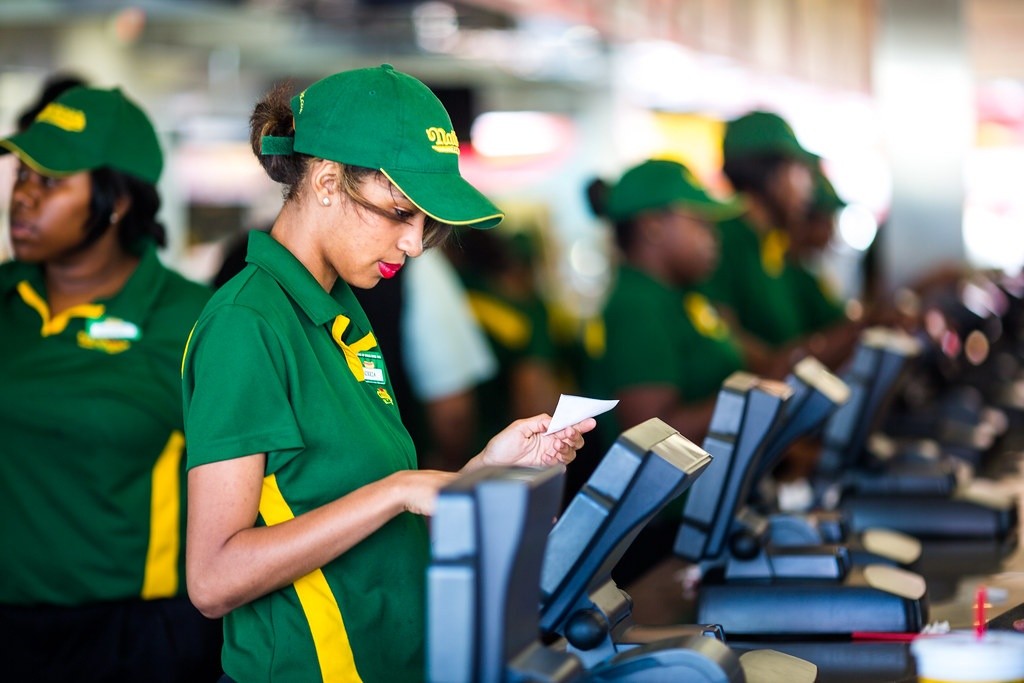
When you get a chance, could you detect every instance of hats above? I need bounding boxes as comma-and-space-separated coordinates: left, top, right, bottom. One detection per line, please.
723, 114, 819, 178
812, 177, 846, 211
603, 159, 748, 226
0, 88, 164, 183
260, 64, 504, 230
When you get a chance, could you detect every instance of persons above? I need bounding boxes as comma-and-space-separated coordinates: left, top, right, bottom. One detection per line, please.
0, 72, 212, 683
177, 64, 597, 683
585, 110, 914, 441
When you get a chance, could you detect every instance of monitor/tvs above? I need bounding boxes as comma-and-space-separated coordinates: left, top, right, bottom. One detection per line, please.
425, 282, 1008, 683
